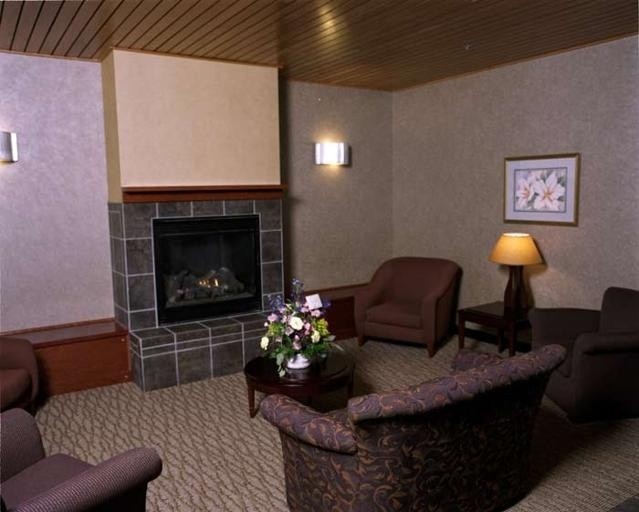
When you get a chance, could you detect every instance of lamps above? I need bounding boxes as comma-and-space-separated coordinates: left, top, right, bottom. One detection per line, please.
315, 142, 349, 165
489, 232, 545, 313
0, 131, 18, 162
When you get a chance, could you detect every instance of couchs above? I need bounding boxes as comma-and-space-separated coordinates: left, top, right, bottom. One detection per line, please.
259, 343, 568, 512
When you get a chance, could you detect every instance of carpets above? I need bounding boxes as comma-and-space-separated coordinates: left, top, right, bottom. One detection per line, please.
26, 334, 639, 512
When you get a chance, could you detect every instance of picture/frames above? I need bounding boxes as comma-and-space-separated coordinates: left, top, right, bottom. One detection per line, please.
503, 152, 580, 226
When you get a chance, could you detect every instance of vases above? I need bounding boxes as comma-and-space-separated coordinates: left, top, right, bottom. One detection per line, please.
284, 352, 312, 370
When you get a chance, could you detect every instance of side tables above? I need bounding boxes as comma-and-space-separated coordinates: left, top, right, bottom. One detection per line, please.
457, 300, 533, 357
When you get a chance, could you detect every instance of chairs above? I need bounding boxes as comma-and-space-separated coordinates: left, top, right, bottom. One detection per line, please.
0, 334, 39, 414
527, 286, 639, 423
0, 408, 162, 512
354, 256, 461, 357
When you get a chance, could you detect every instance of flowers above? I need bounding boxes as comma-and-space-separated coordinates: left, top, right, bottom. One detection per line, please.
260, 277, 336, 379
